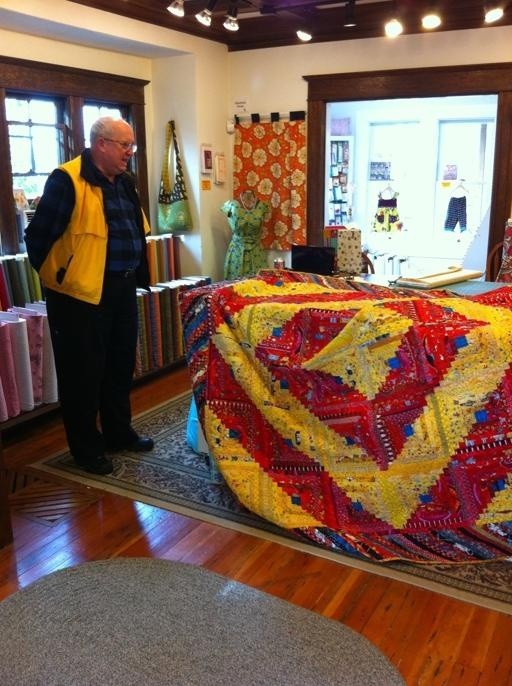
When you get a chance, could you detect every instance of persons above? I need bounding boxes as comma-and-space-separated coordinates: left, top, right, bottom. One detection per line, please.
23, 115, 154, 476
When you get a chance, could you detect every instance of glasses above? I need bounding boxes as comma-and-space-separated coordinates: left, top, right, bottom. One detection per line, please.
105, 138, 138, 152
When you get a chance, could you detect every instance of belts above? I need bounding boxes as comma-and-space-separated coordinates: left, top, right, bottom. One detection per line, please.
105, 268, 132, 280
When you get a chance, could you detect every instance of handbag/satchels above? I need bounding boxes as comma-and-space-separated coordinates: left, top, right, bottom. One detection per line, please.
157, 191, 193, 233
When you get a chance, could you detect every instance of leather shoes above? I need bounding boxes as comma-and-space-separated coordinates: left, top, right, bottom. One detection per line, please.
70, 436, 154, 474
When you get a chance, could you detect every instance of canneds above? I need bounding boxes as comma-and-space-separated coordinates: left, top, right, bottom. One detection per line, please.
273, 259, 285, 269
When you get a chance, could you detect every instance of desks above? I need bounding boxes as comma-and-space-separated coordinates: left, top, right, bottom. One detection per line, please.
178, 266, 512, 499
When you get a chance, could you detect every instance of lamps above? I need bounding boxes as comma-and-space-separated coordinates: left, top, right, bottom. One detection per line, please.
291, 1, 506, 43
165, 0, 244, 32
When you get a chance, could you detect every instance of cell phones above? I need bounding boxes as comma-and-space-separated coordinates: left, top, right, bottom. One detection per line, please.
56, 268, 66, 284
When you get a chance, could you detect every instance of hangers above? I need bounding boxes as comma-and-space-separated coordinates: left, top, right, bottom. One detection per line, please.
378, 180, 400, 199
449, 179, 469, 197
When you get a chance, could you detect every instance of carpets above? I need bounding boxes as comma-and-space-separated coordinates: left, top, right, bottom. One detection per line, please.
1, 556, 410, 686
27, 387, 511, 620
